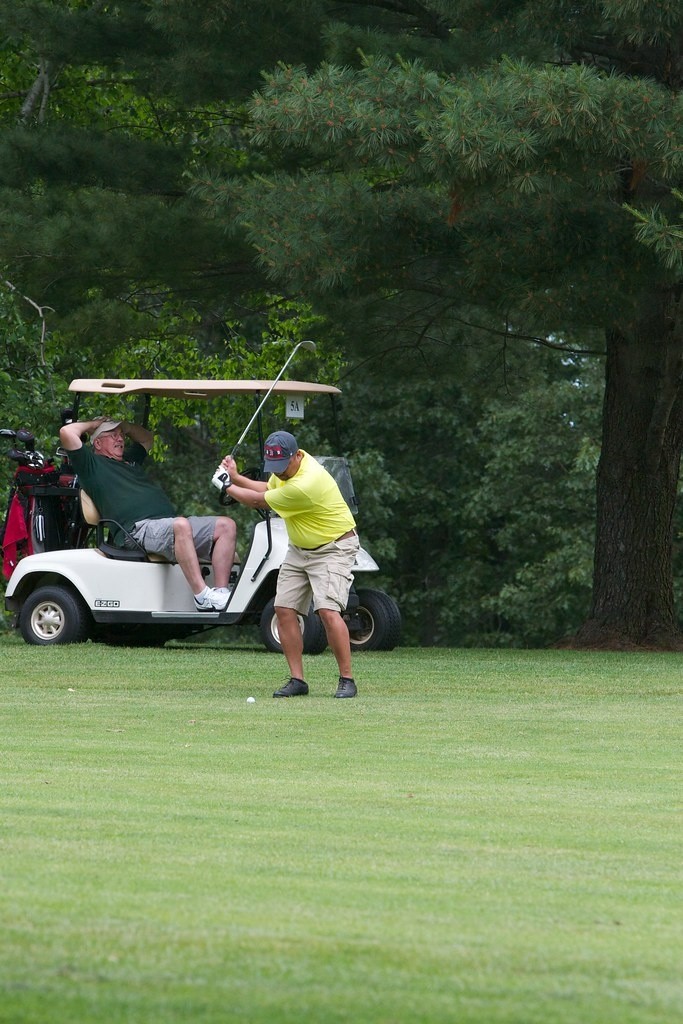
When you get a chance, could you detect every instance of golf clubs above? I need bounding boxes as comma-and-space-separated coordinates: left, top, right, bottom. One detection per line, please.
217, 340, 315, 486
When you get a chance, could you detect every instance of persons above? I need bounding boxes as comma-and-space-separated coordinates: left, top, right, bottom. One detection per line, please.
60, 416, 237, 612
211, 431, 361, 698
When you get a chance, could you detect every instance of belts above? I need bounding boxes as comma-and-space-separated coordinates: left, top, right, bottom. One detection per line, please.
335, 530, 356, 542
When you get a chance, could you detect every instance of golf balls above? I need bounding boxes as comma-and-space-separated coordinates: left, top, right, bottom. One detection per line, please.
247, 697, 256, 703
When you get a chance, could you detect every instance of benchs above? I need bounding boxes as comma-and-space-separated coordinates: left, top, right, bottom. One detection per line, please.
79, 488, 242, 566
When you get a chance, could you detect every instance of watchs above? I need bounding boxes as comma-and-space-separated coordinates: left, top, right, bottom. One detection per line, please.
222, 481, 232, 493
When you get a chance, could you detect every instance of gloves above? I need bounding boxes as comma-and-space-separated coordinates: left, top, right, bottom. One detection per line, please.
211, 465, 230, 491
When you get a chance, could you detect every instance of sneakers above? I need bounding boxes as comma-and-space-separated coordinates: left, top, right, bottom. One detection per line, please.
193, 586, 231, 612
272, 677, 309, 698
333, 674, 357, 698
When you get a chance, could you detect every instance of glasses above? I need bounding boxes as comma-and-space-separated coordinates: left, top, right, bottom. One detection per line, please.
97, 432, 125, 441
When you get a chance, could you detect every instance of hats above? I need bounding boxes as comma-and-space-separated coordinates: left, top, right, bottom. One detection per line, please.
89, 416, 122, 445
263, 430, 299, 474
